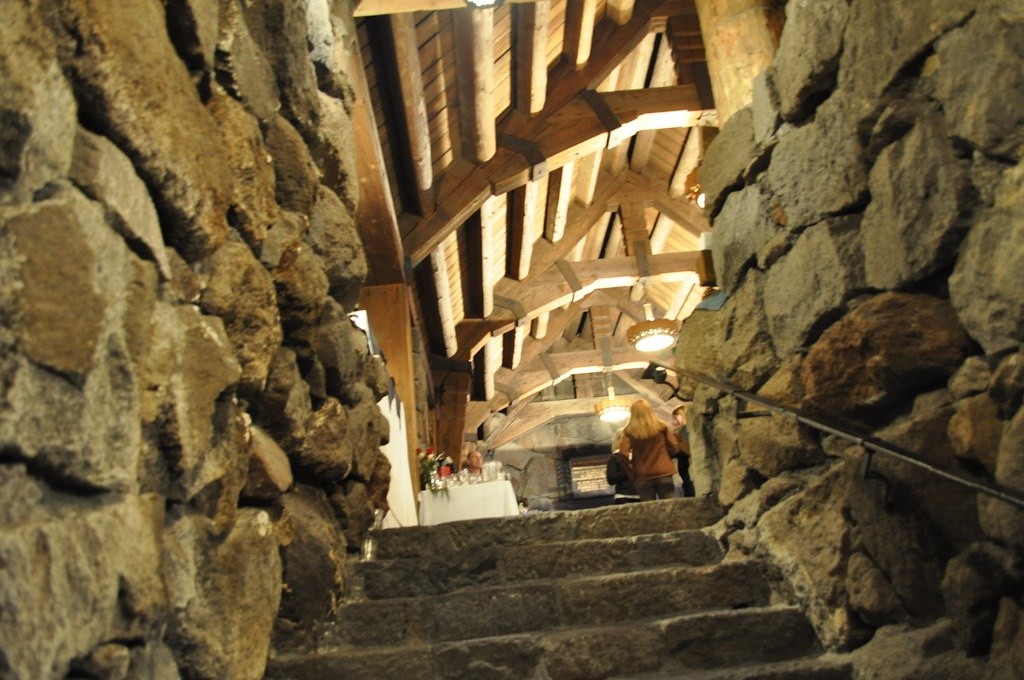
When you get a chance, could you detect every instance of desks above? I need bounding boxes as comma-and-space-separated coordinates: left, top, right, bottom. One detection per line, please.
419, 480, 519, 526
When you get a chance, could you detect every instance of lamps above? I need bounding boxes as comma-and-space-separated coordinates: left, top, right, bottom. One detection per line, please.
594, 371, 633, 422
627, 282, 679, 353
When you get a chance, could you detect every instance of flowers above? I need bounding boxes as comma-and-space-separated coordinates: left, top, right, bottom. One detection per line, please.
416, 448, 454, 476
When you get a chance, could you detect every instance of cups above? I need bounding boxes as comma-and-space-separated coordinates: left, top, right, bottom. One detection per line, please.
374, 509, 383, 528
360, 539, 377, 561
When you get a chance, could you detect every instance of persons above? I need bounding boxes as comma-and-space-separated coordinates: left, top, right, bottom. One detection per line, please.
467, 451, 483, 474
671, 406, 696, 497
606, 427, 641, 504
621, 400, 679, 503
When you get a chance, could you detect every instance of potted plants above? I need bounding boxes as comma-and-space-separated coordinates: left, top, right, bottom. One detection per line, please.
423, 471, 442, 490
